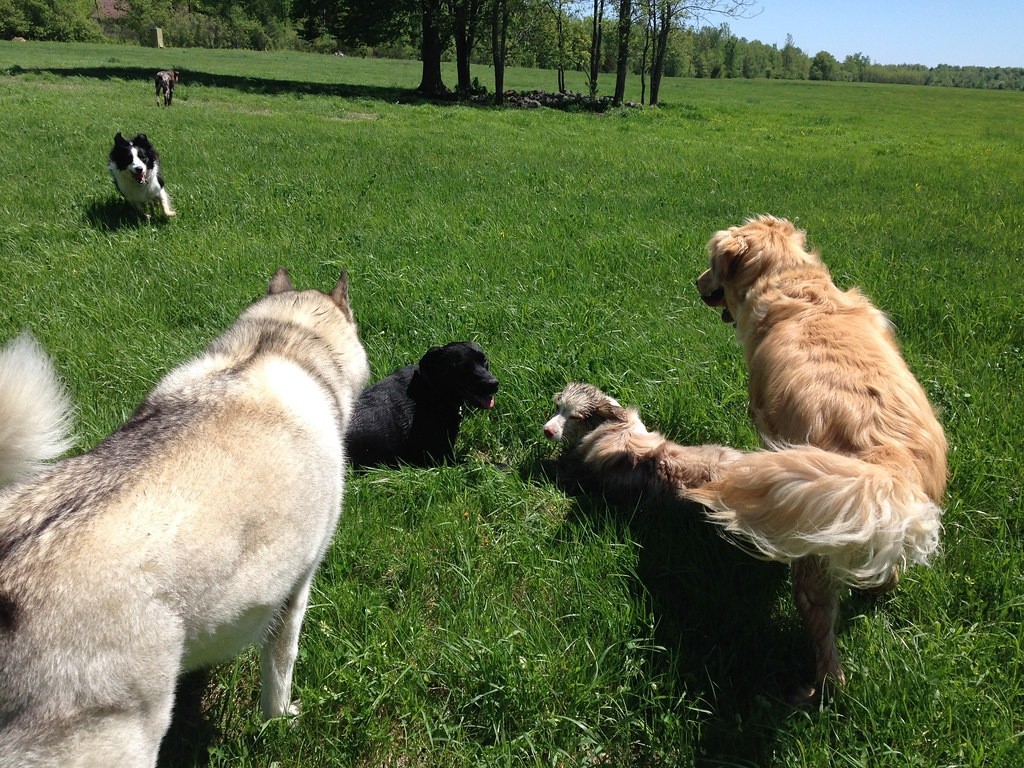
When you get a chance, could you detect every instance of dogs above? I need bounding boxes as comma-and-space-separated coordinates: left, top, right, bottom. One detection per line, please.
542, 212, 953, 707
0, 267, 373, 768
152, 65, 181, 107
107, 131, 177, 227
342, 340, 500, 476
